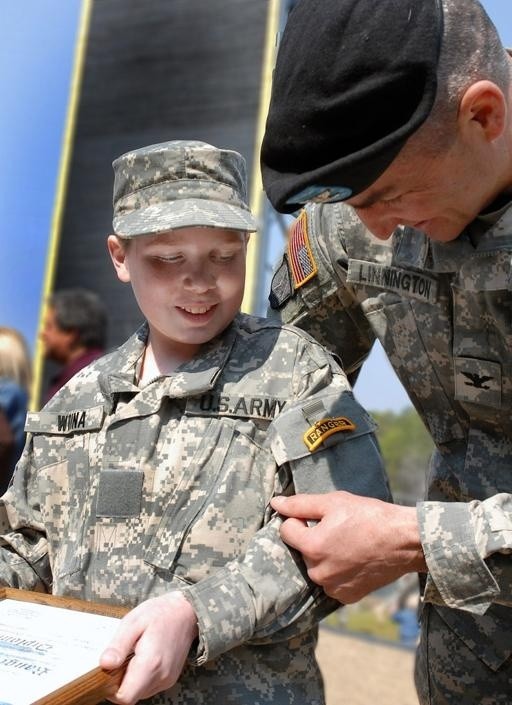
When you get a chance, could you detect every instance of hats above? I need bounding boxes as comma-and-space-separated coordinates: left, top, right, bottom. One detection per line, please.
259, 0, 445, 214
111, 139, 258, 242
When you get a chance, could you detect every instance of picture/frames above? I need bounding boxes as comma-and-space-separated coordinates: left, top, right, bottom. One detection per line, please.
0, 586, 135, 705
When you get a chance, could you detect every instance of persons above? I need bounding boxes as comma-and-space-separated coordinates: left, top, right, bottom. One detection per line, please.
0, 140, 392, 705
0, 288, 111, 489
261, 0, 512, 705
